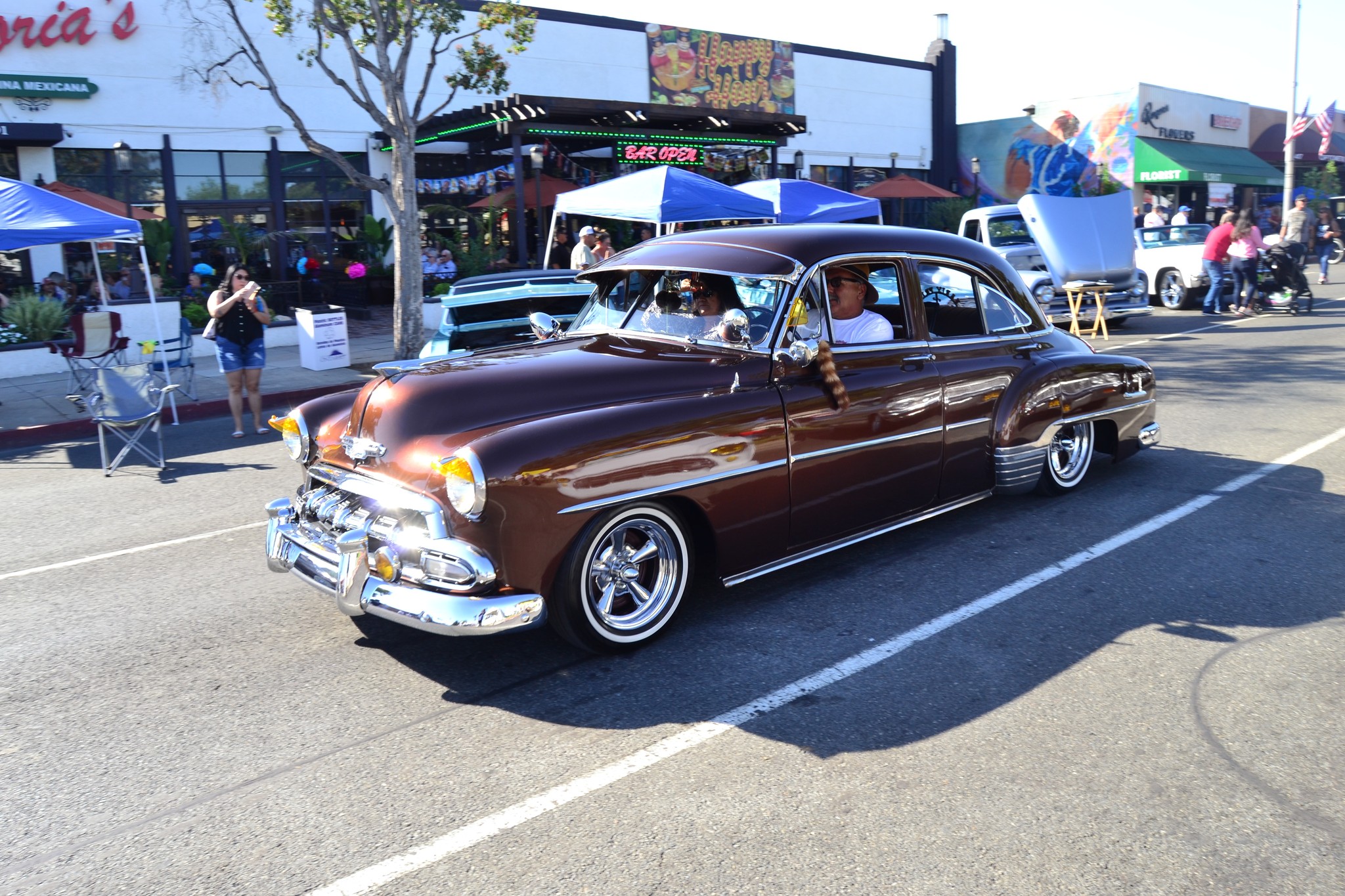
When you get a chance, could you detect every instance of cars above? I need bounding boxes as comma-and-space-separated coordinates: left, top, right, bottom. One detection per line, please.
932, 190, 1154, 327
268, 222, 1159, 661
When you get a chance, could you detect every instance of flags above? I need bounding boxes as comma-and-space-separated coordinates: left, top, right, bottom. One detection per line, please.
416, 138, 608, 194
1315, 100, 1335, 156
1283, 97, 1310, 144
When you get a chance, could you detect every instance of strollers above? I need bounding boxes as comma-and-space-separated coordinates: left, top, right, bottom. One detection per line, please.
1254, 239, 1312, 316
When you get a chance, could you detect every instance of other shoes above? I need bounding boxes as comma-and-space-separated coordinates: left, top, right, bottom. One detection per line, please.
1247, 308, 1261, 313
1317, 278, 1329, 284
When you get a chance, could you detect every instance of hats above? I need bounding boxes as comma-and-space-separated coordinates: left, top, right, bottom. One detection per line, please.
1178, 206, 1192, 211
834, 263, 879, 305
39, 277, 56, 287
1295, 194, 1308, 201
578, 226, 601, 237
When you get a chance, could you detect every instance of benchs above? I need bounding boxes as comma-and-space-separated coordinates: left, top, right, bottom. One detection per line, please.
871, 301, 1009, 339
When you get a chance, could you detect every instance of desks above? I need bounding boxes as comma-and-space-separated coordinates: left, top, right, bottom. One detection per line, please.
1062, 284, 1116, 341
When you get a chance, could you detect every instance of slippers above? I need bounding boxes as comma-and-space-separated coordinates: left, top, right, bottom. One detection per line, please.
232, 431, 245, 438
256, 428, 270, 434
1237, 310, 1254, 317
1228, 305, 1238, 312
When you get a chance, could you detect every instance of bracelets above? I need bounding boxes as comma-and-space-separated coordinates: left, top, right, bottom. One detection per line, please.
247, 305, 258, 313
1280, 237, 1284, 239
1309, 237, 1316, 239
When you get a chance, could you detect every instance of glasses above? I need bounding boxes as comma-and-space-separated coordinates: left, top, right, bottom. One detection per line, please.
825, 277, 862, 288
1318, 211, 1327, 214
120, 273, 131, 277
441, 255, 449, 257
1298, 200, 1309, 202
693, 287, 719, 299
588, 233, 597, 238
232, 273, 250, 281
427, 256, 432, 258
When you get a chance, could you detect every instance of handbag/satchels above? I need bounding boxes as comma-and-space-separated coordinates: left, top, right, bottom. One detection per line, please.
202, 317, 221, 341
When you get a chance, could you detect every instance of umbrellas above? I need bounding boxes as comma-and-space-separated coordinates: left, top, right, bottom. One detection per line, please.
469, 172, 583, 247
37, 181, 167, 305
852, 173, 962, 226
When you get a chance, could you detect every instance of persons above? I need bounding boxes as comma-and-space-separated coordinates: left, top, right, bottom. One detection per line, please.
41, 259, 211, 337
206, 262, 270, 438
423, 194, 1345, 349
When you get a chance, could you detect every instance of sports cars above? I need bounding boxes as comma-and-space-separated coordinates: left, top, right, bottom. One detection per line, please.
1133, 224, 1274, 310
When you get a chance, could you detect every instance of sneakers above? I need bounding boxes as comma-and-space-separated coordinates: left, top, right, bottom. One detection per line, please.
1201, 310, 1223, 317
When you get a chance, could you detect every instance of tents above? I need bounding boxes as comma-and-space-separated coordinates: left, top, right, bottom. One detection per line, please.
0, 175, 180, 426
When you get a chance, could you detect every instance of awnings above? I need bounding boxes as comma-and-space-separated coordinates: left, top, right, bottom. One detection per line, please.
1133, 135, 1284, 187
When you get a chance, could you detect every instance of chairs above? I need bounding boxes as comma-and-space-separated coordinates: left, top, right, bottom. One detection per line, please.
43, 311, 132, 395
84, 363, 178, 479
138, 317, 198, 403
